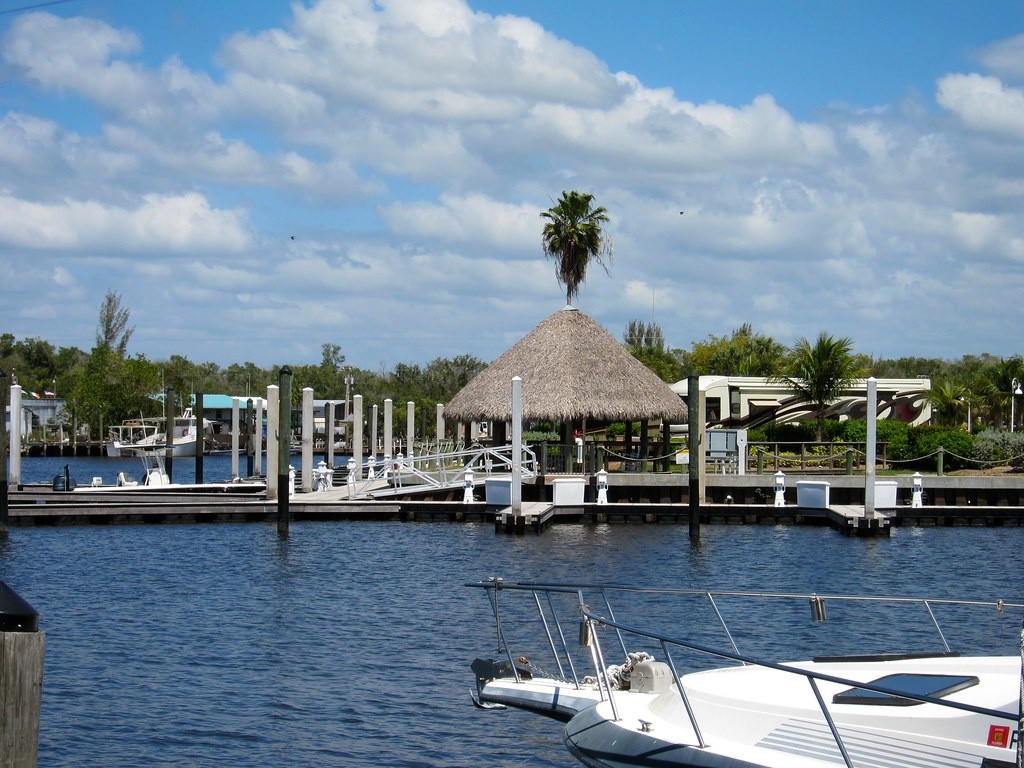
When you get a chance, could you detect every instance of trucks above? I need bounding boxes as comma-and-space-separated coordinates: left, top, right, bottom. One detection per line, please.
659, 375, 931, 438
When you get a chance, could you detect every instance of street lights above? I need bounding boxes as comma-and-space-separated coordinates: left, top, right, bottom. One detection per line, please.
1011, 377, 1023, 433
960, 388, 971, 433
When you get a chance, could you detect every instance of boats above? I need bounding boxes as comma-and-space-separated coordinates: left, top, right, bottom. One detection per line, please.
113, 408, 210, 457
72, 441, 267, 494
465, 576, 1024, 768
